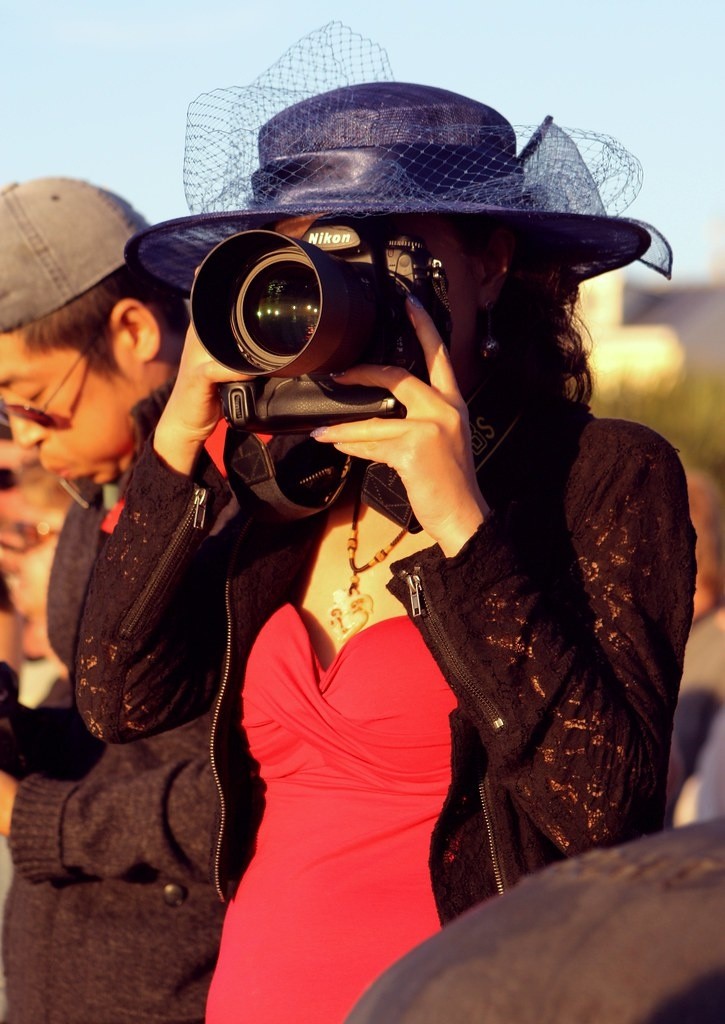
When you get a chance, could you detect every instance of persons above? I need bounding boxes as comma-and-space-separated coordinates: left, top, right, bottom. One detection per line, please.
74, 21, 699, 1023
0, 177, 725, 1024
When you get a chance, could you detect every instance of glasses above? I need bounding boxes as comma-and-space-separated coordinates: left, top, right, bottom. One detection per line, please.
0, 319, 108, 427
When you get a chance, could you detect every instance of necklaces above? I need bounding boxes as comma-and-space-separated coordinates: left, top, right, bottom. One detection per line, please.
323, 492, 407, 642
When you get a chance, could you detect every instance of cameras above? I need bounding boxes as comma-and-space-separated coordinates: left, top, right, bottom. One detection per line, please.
190, 227, 449, 400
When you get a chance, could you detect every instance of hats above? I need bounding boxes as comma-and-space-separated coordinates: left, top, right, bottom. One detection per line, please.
124, 84, 673, 296
0, 178, 151, 331
